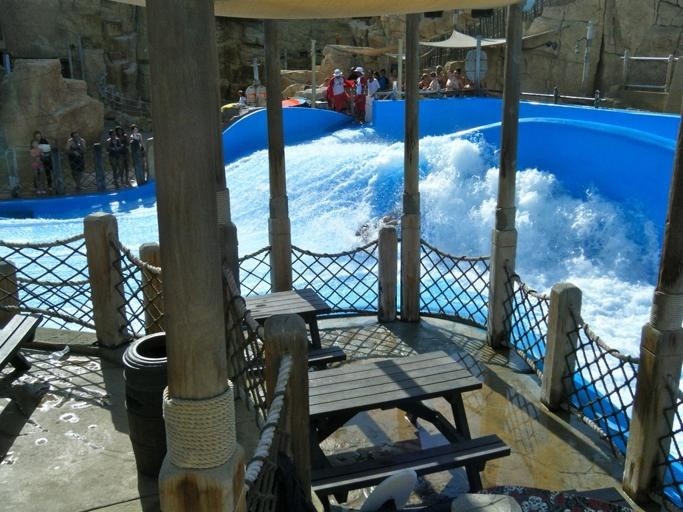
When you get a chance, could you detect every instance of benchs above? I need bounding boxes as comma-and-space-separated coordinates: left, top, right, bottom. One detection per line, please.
247, 349, 344, 380
310, 433, 511, 502
0, 313, 44, 372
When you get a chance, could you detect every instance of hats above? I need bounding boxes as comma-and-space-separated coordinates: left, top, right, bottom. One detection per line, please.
354, 67, 365, 75
333, 69, 342, 76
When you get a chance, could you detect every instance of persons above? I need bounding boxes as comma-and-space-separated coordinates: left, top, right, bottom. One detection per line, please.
128, 123, 147, 186
29, 139, 48, 195
323, 65, 390, 124
105, 128, 125, 188
31, 130, 54, 191
64, 129, 86, 190
418, 64, 474, 98
114, 125, 129, 186
238, 89, 247, 106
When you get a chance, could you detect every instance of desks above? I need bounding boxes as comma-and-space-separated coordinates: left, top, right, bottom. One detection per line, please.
243, 284, 332, 349
300, 351, 482, 455
